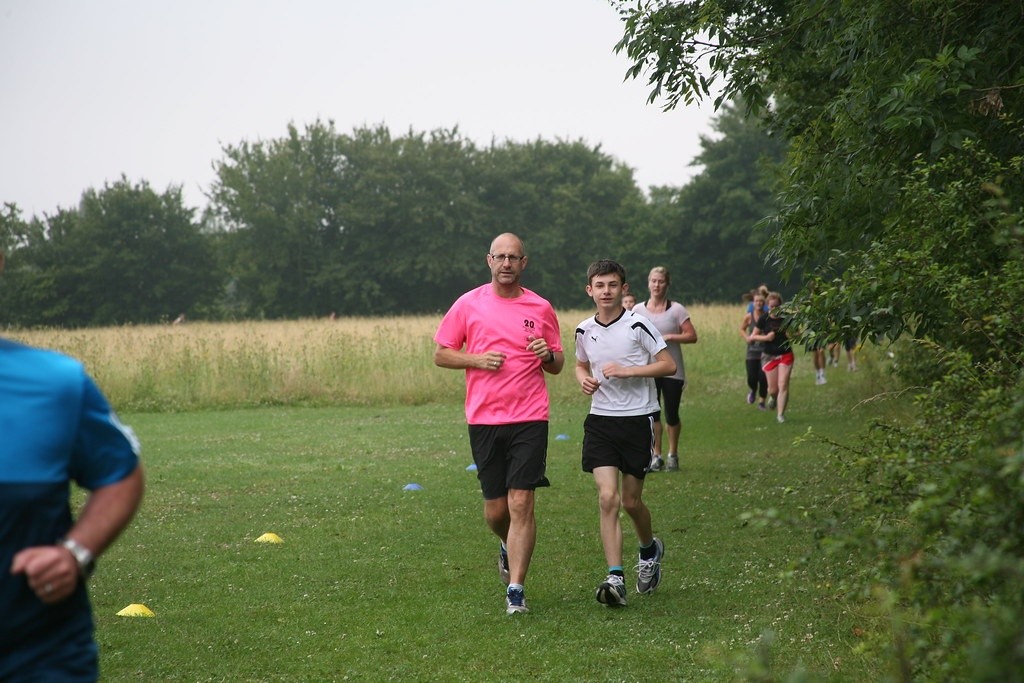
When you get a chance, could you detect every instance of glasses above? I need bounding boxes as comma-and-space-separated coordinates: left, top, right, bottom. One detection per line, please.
490, 254, 525, 263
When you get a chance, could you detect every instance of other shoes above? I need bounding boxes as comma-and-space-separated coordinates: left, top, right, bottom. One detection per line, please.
815, 374, 828, 385
777, 413, 787, 423
846, 362, 859, 373
766, 396, 777, 408
747, 386, 757, 404
827, 357, 838, 367
757, 401, 768, 412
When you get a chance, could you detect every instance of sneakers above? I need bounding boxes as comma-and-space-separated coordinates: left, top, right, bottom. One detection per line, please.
634, 536, 666, 596
503, 583, 530, 616
595, 573, 627, 608
663, 454, 679, 470
497, 541, 512, 587
650, 456, 666, 472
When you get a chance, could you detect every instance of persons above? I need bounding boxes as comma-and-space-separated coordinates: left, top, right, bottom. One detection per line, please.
844, 336, 857, 371
739, 294, 768, 411
632, 267, 697, 472
750, 292, 799, 423
0, 337, 145, 683
621, 293, 635, 311
432, 233, 565, 614
827, 342, 842, 368
813, 347, 828, 386
748, 286, 769, 313
573, 259, 677, 608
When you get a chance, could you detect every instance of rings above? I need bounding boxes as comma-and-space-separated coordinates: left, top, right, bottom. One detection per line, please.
45, 583, 53, 595
494, 362, 496, 365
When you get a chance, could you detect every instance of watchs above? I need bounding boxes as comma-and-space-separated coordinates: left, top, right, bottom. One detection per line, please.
61, 538, 95, 579
542, 350, 555, 363
